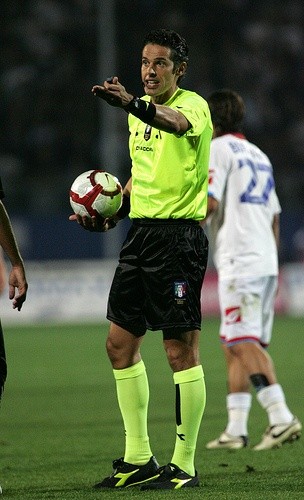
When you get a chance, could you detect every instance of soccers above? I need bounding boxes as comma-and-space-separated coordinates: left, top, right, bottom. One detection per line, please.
70, 169, 123, 222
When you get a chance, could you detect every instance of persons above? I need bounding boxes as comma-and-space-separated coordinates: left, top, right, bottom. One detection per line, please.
0, 179, 28, 495
69, 30, 213, 490
200, 90, 302, 451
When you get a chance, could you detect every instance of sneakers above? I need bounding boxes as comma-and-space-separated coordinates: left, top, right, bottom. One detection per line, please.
92, 456, 163, 489
253, 416, 301, 451
206, 432, 248, 450
140, 463, 200, 489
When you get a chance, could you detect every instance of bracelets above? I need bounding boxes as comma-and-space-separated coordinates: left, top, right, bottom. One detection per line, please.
125, 97, 156, 124
